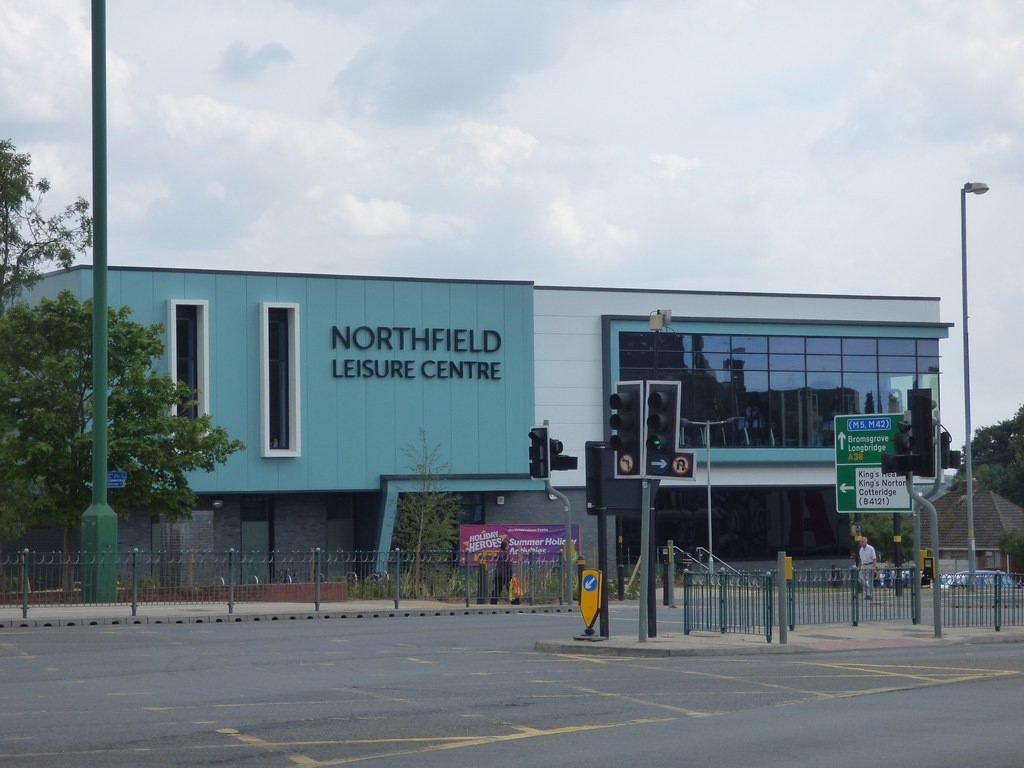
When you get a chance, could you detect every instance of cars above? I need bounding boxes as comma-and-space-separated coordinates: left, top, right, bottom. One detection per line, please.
868, 570, 1021, 588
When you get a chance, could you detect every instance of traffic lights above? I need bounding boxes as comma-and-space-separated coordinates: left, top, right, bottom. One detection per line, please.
607, 380, 642, 478
644, 379, 680, 477
527, 426, 551, 480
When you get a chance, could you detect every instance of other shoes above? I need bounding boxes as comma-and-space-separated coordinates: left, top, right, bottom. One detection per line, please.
865, 596, 872, 600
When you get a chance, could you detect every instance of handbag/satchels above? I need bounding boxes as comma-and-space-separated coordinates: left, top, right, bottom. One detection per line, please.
508, 579, 516, 602
513, 577, 524, 598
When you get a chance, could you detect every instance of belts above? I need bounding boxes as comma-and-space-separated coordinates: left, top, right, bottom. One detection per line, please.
864, 563, 873, 565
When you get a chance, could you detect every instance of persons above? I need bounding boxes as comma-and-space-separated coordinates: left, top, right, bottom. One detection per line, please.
831, 565, 845, 587
873, 565, 892, 589
490, 541, 521, 605
854, 537, 877, 600
